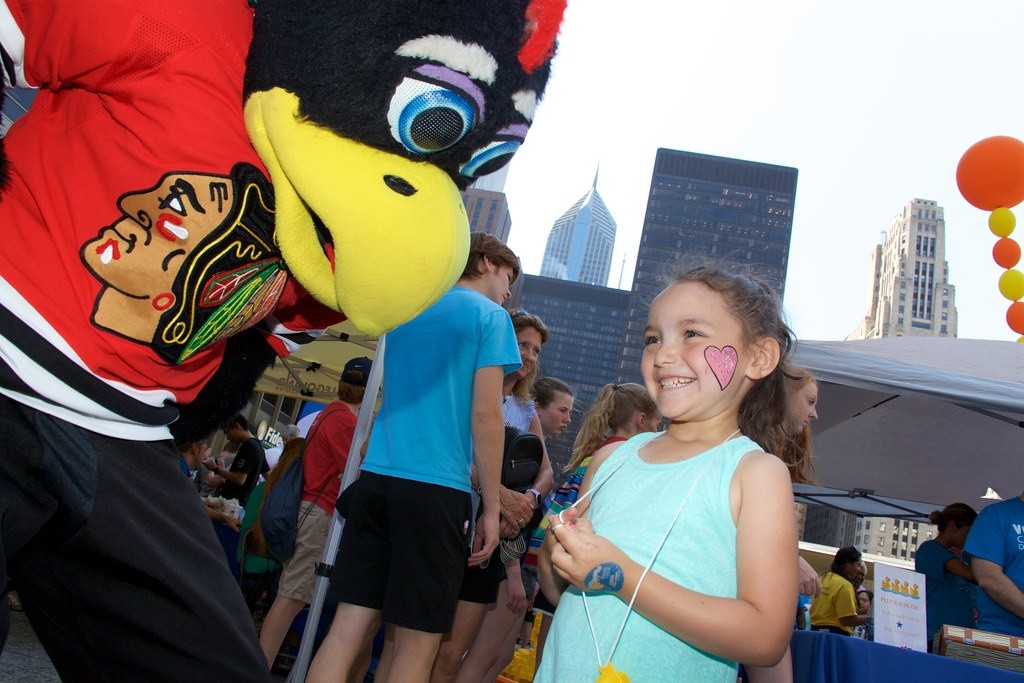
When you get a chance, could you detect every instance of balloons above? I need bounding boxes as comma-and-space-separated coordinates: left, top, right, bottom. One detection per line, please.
956, 135, 1024, 342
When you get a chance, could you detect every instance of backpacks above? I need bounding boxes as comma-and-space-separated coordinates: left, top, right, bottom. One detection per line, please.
480, 425, 544, 569
260, 408, 349, 563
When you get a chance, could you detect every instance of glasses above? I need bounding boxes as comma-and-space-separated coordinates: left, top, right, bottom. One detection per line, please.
511, 308, 534, 319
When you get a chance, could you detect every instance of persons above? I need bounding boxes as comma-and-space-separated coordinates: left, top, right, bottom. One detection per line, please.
809, 494, 1024, 652
184, 231, 822, 683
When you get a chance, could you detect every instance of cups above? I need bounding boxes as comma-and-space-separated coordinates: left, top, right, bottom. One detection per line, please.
228, 504, 244, 520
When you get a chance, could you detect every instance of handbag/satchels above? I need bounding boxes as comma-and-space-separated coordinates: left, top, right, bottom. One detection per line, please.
533, 456, 592, 539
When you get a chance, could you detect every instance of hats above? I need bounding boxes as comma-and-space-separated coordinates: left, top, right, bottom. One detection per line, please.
341, 357, 381, 391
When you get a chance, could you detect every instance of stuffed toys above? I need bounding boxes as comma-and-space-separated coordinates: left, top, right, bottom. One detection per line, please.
0, 0, 562, 683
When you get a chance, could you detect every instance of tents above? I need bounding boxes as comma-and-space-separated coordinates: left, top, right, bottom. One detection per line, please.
253, 320, 382, 683
783, 336, 1024, 524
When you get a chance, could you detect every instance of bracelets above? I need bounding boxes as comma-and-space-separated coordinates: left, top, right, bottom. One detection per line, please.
214, 466, 219, 475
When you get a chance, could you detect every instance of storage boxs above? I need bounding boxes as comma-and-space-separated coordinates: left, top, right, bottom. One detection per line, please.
937, 625, 1024, 674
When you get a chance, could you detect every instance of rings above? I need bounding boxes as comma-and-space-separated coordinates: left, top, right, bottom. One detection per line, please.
512, 528, 517, 534
518, 518, 524, 522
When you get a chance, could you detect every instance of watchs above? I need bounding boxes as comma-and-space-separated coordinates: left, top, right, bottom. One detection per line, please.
526, 488, 542, 509
